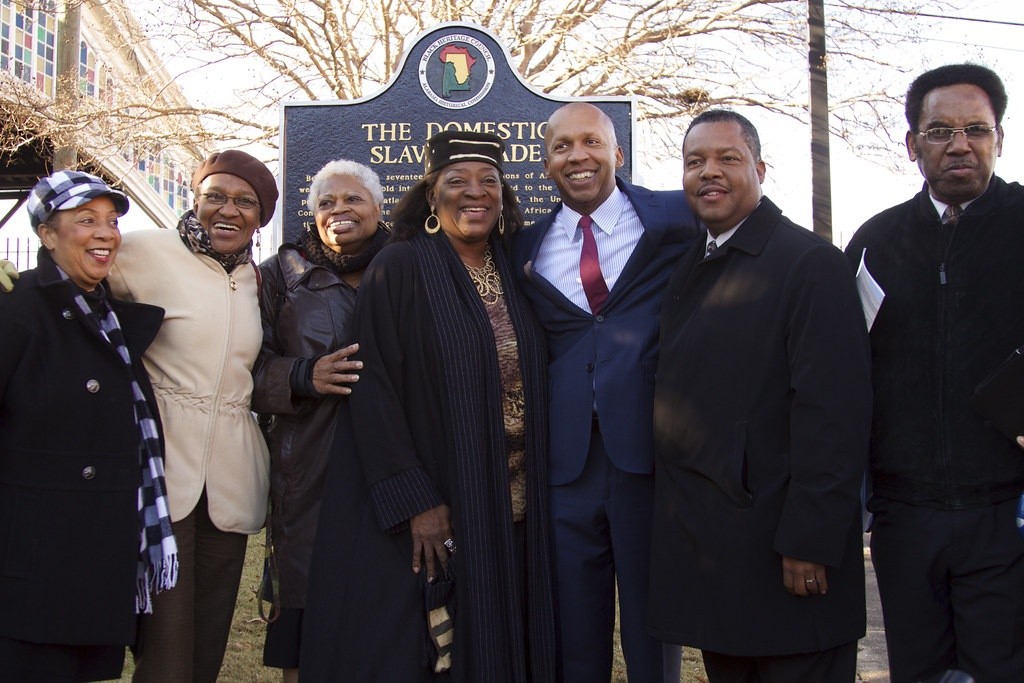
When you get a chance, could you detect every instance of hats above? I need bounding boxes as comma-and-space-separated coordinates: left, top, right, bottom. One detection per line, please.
191, 150, 279, 227
424, 130, 505, 175
26, 170, 129, 240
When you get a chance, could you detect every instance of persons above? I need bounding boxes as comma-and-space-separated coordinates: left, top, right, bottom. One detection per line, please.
503, 103, 701, 682
298, 134, 555, 683
249, 159, 393, 683
647, 109, 875, 683
0, 172, 166, 683
844, 65, 1024, 683
0, 151, 270, 683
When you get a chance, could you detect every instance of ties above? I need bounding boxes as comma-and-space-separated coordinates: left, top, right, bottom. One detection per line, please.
942, 206, 962, 226
704, 241, 715, 258
578, 215, 610, 315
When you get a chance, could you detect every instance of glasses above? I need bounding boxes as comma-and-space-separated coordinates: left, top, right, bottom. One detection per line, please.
199, 192, 262, 209
917, 125, 998, 144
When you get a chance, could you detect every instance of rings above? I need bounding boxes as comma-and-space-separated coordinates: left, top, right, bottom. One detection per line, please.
806, 577, 815, 583
443, 539, 456, 554
